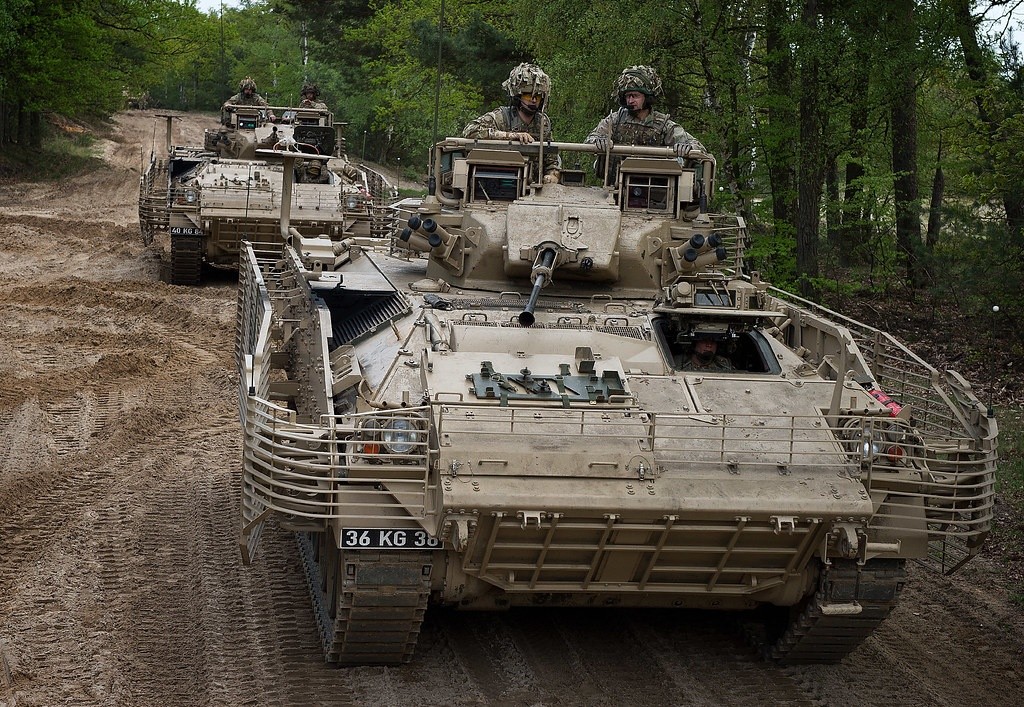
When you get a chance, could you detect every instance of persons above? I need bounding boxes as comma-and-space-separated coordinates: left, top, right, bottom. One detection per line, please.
674, 324, 737, 374
299, 81, 329, 109
462, 63, 562, 184
225, 76, 276, 122
583, 66, 707, 186
294, 157, 329, 183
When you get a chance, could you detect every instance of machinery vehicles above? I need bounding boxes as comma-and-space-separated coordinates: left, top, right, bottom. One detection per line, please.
134, 90, 403, 286
234, 101, 1000, 670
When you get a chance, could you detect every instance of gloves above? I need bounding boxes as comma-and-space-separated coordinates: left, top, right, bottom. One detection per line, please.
544, 170, 559, 184
508, 132, 535, 144
596, 137, 614, 150
674, 142, 692, 156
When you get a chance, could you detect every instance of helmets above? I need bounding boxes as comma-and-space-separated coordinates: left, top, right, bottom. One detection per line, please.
239, 77, 257, 92
502, 63, 551, 97
301, 83, 321, 96
618, 65, 664, 107
